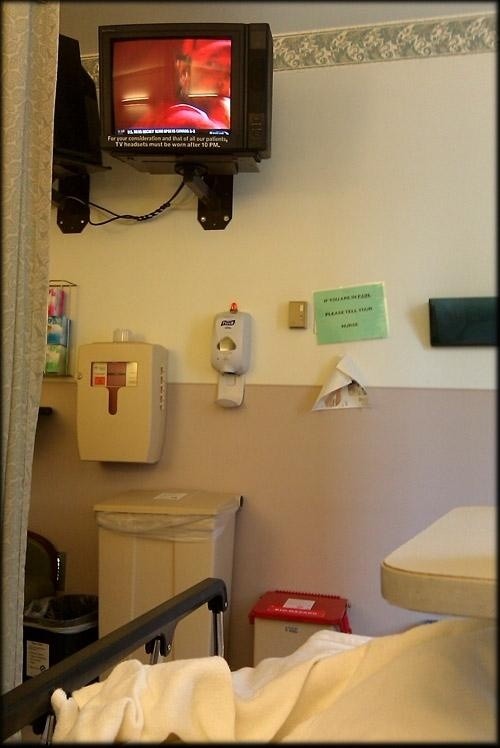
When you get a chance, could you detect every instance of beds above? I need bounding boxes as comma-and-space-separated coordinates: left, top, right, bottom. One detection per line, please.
1, 578, 500, 748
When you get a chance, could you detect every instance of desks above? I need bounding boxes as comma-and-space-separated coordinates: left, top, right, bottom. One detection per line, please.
381, 506, 500, 619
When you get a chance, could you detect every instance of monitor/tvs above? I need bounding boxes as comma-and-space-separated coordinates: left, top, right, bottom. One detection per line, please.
53, 34, 102, 166
99, 25, 273, 162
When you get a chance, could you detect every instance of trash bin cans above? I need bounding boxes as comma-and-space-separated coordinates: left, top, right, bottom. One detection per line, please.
249, 590, 352, 667
24, 529, 101, 682
94, 491, 243, 681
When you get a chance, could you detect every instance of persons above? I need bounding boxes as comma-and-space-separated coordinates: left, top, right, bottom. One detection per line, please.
129, 38, 232, 129
